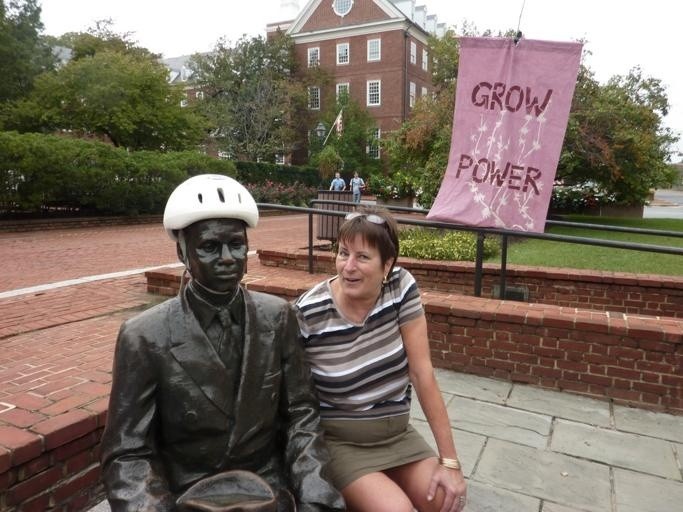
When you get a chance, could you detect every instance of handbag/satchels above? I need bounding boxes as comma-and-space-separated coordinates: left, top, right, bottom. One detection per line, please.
360, 188, 366, 194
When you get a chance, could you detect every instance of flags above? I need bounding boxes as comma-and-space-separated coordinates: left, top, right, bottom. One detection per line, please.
335, 115, 344, 139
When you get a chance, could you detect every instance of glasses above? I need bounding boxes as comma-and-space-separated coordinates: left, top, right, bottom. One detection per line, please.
342, 211, 386, 225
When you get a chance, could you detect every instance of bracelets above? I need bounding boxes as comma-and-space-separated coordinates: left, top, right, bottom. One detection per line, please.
438, 458, 461, 469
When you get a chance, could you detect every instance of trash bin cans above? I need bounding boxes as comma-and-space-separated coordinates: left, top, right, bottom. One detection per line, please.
317, 190, 355, 245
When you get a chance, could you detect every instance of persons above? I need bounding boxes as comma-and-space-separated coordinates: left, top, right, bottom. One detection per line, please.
350, 171, 366, 202
98, 173, 347, 512
330, 172, 347, 191
290, 205, 467, 512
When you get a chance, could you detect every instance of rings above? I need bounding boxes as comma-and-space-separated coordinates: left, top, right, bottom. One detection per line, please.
458, 495, 465, 506
